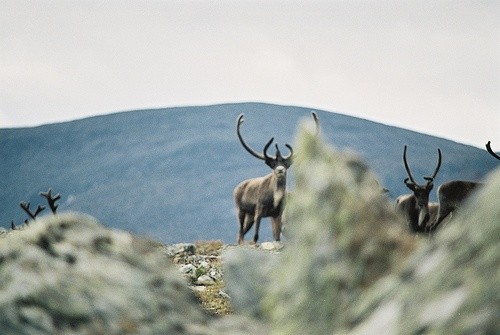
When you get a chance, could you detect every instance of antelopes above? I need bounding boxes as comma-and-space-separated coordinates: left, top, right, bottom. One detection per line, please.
425, 141, 500, 239
233, 111, 321, 247
395, 144, 443, 236
